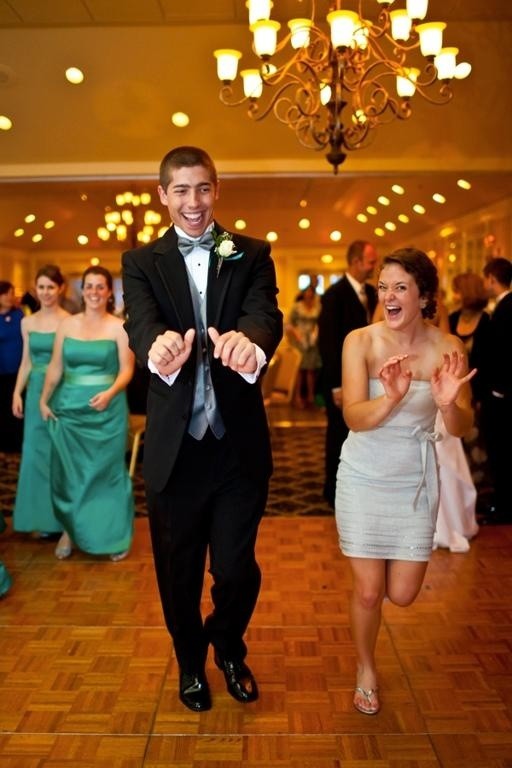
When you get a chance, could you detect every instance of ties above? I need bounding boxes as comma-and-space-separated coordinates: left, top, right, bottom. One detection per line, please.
360, 287, 371, 325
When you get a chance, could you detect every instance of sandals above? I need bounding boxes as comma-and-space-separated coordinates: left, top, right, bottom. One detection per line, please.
111, 552, 128, 561
55, 547, 72, 559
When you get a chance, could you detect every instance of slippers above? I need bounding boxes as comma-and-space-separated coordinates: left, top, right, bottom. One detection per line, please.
353, 687, 379, 714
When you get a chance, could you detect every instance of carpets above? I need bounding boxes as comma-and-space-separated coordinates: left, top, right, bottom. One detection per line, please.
0, 428, 486, 517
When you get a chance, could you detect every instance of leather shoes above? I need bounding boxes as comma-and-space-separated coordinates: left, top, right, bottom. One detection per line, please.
214, 650, 259, 703
178, 663, 211, 711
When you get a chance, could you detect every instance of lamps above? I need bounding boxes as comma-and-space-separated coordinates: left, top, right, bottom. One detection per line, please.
211, 0, 472, 176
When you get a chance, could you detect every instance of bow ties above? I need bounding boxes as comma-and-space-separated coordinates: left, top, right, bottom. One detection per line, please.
176, 232, 216, 264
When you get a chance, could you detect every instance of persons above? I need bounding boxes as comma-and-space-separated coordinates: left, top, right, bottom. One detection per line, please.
335, 247, 476, 713
122, 147, 284, 713
0, 265, 147, 596
285, 241, 380, 511
424, 256, 512, 526
